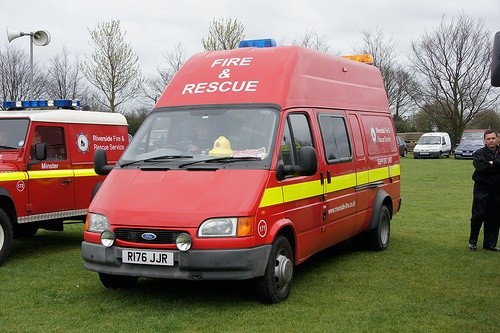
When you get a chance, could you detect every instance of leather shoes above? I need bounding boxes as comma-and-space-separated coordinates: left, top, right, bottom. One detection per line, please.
483, 245, 500, 251
469, 243, 477, 250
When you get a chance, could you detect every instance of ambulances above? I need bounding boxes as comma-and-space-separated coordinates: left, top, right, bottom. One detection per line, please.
0, 100, 129, 267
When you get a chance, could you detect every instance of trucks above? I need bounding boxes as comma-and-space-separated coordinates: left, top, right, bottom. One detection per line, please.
79, 39, 403, 304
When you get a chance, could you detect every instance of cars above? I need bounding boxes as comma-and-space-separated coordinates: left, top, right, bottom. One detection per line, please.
454, 139, 485, 159
396, 135, 408, 158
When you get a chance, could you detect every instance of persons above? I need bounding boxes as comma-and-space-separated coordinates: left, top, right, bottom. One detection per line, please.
27, 128, 61, 160
468, 129, 500, 251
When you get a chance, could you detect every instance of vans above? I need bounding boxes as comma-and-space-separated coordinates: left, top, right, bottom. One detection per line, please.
412, 131, 452, 159
